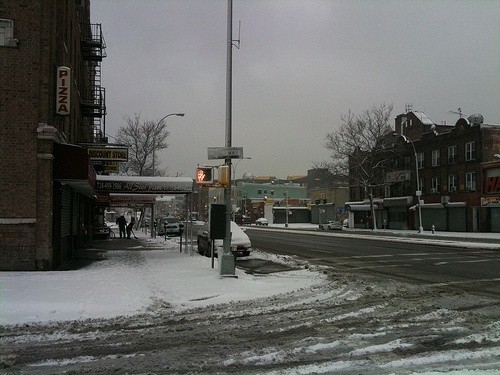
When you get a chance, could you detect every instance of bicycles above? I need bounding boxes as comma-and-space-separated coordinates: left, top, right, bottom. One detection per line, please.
103, 222, 116, 240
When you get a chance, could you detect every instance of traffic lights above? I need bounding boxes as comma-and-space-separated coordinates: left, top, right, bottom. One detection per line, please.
218, 166, 230, 185
195, 167, 214, 185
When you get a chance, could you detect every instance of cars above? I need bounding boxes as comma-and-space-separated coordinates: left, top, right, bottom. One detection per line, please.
197, 221, 252, 258
140, 218, 151, 227
321, 221, 343, 231
255, 217, 268, 226
158, 216, 180, 236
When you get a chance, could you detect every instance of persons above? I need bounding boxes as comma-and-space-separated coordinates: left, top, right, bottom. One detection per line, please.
126, 217, 136, 239
116, 216, 127, 239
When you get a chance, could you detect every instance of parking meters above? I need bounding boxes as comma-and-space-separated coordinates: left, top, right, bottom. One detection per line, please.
178, 222, 184, 253
154, 221, 158, 239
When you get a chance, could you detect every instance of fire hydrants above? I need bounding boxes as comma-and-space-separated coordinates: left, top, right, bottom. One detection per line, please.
431, 225, 435, 234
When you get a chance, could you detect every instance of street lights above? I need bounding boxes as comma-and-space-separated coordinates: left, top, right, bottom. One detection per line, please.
392, 132, 424, 233
232, 157, 252, 223
151, 113, 185, 238
237, 175, 258, 215
